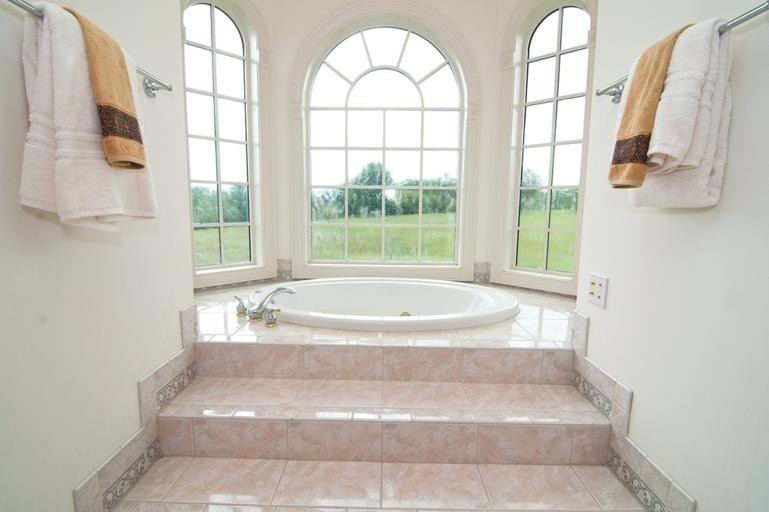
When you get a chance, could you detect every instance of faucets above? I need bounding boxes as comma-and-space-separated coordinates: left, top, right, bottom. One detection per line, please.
246, 286, 297, 322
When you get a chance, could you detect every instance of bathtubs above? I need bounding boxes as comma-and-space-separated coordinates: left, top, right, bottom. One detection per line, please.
249, 277, 521, 332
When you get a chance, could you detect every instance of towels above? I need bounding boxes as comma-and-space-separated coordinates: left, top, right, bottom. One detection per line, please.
608, 17, 736, 211
22, 4, 156, 234
